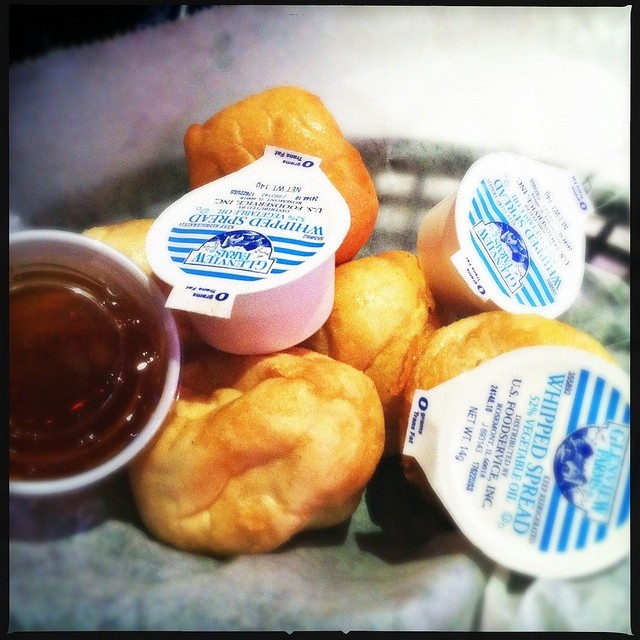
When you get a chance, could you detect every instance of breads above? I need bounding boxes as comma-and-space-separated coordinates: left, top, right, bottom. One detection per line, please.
80, 218, 214, 362
185, 87, 379, 266
400, 312, 621, 503
297, 249, 456, 429
129, 347, 386, 558
